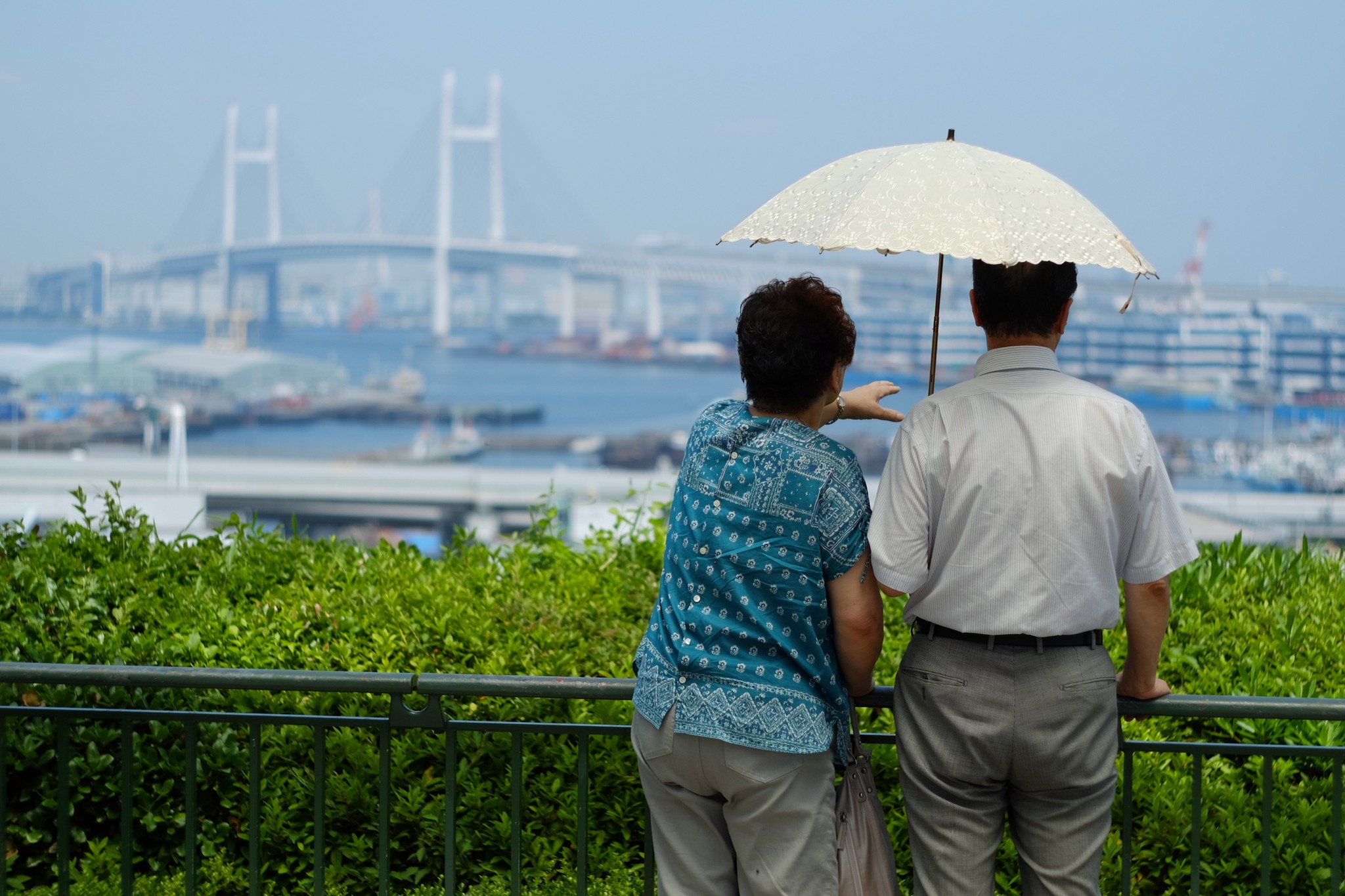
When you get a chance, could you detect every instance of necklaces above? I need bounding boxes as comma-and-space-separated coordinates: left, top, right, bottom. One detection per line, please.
752, 404, 807, 426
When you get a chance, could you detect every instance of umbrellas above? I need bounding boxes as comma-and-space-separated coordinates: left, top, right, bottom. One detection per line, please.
716, 129, 1161, 395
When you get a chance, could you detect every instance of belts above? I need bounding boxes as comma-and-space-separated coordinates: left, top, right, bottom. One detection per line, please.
914, 616, 1105, 650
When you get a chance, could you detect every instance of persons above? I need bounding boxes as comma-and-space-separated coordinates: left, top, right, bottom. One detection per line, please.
631, 276, 904, 896
867, 261, 1199, 896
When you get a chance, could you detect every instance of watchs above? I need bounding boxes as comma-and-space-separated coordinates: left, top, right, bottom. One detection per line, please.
826, 394, 845, 425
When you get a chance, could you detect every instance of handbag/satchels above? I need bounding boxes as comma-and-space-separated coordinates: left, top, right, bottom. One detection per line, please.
834, 693, 898, 896
